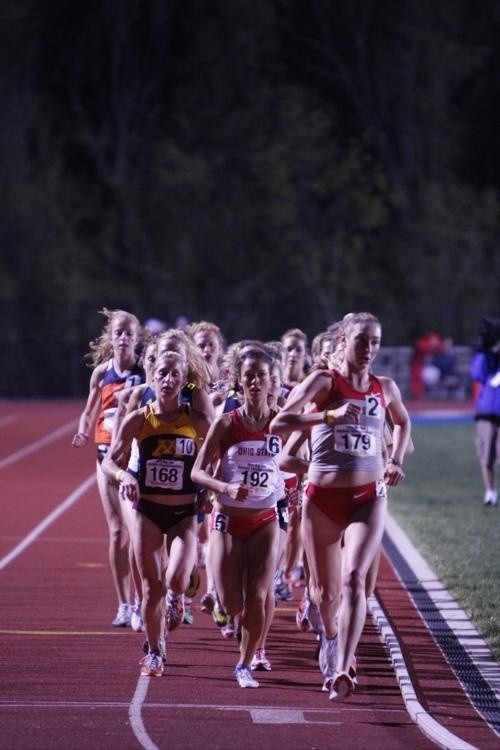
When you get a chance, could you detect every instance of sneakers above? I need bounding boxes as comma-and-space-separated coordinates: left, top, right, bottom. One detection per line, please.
200, 593, 241, 642
483, 489, 498, 507
296, 587, 324, 632
319, 634, 358, 702
234, 668, 260, 688
140, 641, 166, 677
251, 648, 271, 671
112, 565, 198, 631
273, 563, 307, 602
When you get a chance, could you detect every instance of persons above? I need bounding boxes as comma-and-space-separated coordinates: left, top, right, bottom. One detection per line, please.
403, 329, 468, 403
71, 306, 414, 701
470, 333, 500, 508
